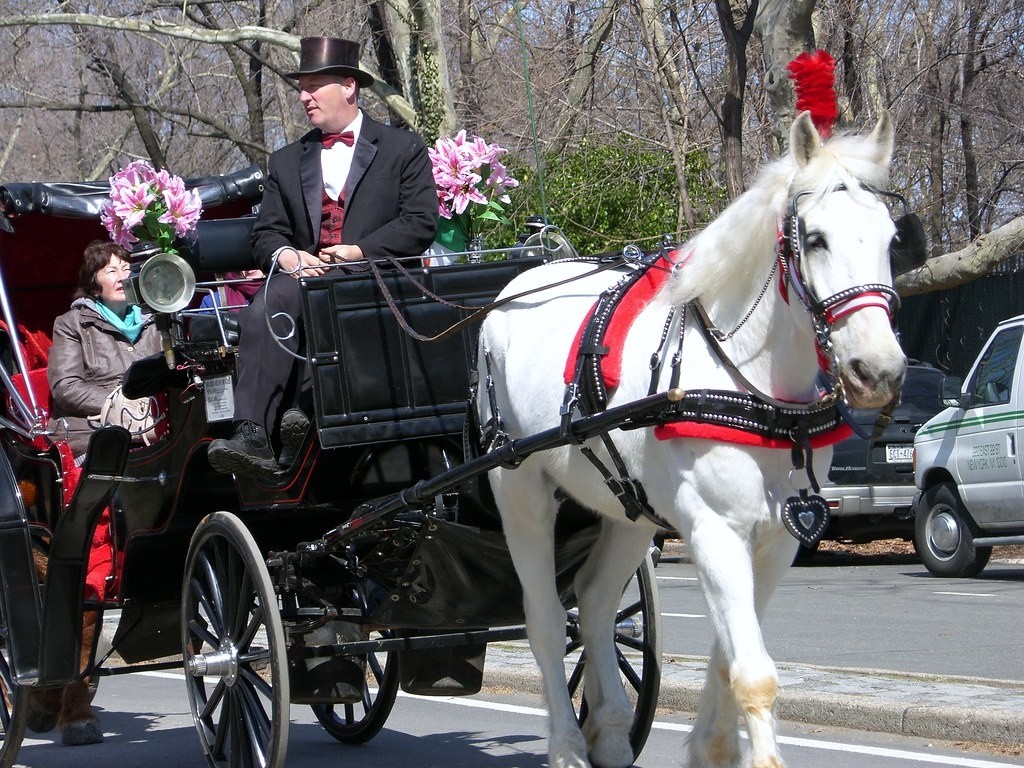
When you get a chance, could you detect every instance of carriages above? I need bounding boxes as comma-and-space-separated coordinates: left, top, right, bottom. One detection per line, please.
0, 64, 909, 768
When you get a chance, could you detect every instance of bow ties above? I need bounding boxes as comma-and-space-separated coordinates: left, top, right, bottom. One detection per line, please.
321, 131, 354, 149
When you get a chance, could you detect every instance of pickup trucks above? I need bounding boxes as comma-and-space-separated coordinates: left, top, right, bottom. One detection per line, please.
906, 304, 1023, 582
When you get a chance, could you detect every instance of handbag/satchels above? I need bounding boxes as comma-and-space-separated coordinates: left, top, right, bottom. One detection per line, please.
100, 385, 158, 443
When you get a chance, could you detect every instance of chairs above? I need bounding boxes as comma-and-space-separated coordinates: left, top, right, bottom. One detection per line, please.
173, 216, 261, 348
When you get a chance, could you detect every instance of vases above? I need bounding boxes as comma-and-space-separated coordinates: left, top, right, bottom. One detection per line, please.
464, 241, 485, 264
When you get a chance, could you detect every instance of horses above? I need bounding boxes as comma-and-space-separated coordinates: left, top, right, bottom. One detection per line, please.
476, 104, 908, 768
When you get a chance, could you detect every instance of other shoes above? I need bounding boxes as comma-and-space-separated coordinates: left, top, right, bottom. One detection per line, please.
209, 420, 278, 477
280, 408, 311, 465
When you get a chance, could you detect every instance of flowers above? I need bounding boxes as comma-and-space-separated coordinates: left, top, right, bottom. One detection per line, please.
99, 159, 204, 255
427, 127, 520, 253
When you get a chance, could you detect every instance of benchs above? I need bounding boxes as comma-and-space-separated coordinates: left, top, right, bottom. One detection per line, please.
6, 365, 82, 510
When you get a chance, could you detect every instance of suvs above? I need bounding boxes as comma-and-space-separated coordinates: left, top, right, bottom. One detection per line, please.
648, 341, 960, 561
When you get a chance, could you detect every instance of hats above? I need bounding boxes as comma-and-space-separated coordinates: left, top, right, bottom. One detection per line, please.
284, 37, 374, 87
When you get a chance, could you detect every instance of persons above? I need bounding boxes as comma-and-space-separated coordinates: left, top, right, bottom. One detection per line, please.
198, 213, 267, 316
50, 241, 167, 548
210, 38, 438, 484
